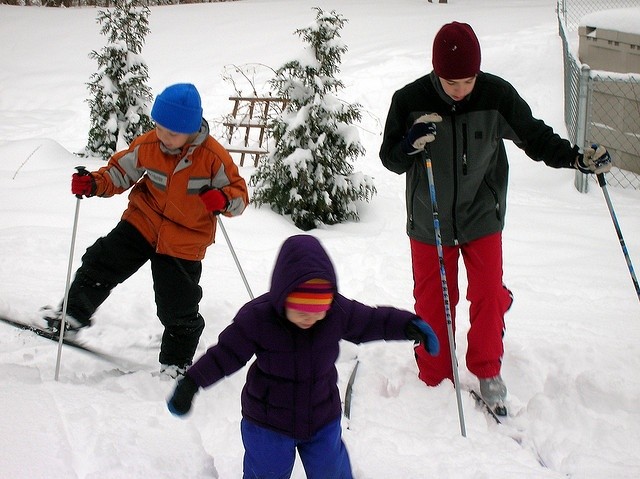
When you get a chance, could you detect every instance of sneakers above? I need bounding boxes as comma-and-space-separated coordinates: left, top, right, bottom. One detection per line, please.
159, 363, 193, 382
37, 302, 86, 333
480, 376, 507, 406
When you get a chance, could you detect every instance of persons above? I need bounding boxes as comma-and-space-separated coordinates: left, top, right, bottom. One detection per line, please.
378, 18, 613, 417
43, 82, 249, 379
166, 233, 442, 479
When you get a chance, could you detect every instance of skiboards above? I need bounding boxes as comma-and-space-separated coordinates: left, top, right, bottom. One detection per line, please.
468, 390, 548, 469
0, 312, 155, 374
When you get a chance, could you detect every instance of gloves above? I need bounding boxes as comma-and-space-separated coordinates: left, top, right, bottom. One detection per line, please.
400, 112, 443, 157
71, 173, 91, 198
573, 143, 612, 174
169, 376, 201, 415
407, 316, 441, 357
199, 188, 227, 213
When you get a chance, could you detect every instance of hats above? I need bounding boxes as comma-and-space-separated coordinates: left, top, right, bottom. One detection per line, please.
432, 21, 481, 80
151, 83, 203, 134
284, 278, 335, 313
